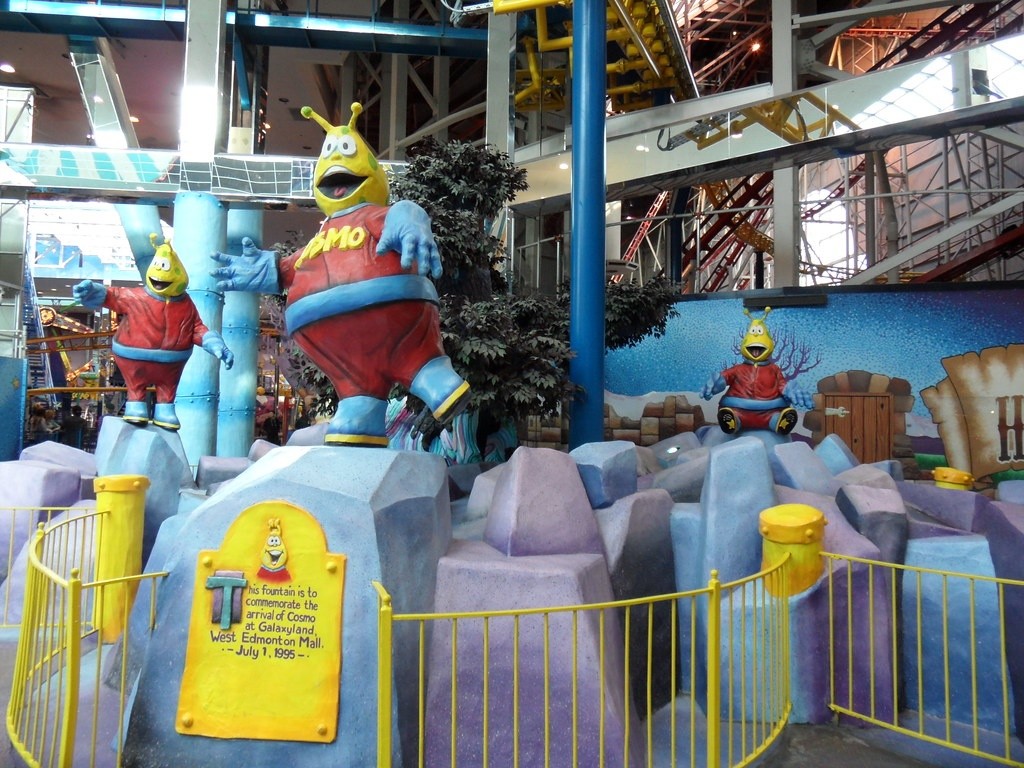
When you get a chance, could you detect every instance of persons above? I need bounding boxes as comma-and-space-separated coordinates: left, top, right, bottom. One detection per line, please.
262, 409, 282, 447
29, 401, 117, 450
294, 406, 313, 431
